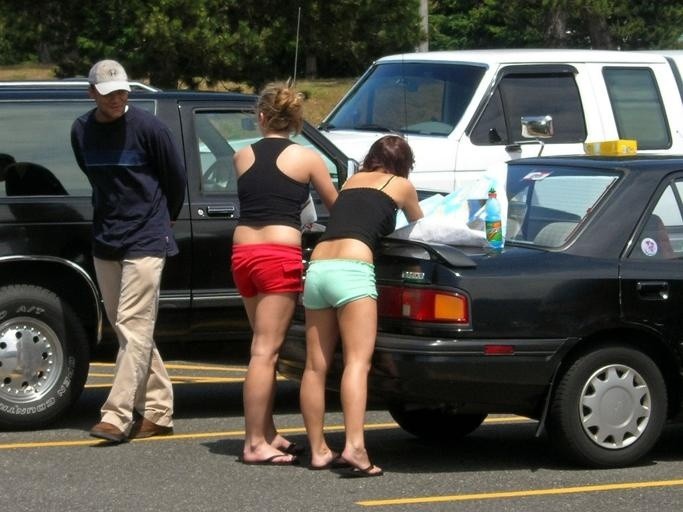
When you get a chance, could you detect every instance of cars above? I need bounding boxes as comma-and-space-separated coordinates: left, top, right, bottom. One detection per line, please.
276, 151, 683, 467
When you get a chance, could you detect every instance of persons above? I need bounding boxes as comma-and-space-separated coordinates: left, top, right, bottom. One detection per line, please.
231, 80, 339, 465
69, 58, 190, 443
299, 133, 423, 479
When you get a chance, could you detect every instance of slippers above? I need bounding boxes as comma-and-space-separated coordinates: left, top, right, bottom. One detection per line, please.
309, 451, 351, 470
339, 463, 384, 476
244, 451, 299, 465
279, 442, 304, 454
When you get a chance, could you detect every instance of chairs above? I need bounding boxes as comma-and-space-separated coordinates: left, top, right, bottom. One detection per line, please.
635, 213, 674, 261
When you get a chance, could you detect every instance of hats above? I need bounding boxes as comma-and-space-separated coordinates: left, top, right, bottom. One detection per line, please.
89, 60, 132, 96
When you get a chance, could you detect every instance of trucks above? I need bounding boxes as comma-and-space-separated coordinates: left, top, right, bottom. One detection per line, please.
197, 46, 683, 254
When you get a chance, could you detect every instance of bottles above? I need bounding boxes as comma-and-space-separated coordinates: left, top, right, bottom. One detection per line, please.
484, 186, 504, 249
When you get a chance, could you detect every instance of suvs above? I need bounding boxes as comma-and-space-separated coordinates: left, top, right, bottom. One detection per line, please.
0, 77, 449, 430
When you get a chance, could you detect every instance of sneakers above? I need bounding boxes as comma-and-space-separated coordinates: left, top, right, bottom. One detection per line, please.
90, 423, 128, 443
128, 415, 172, 438
